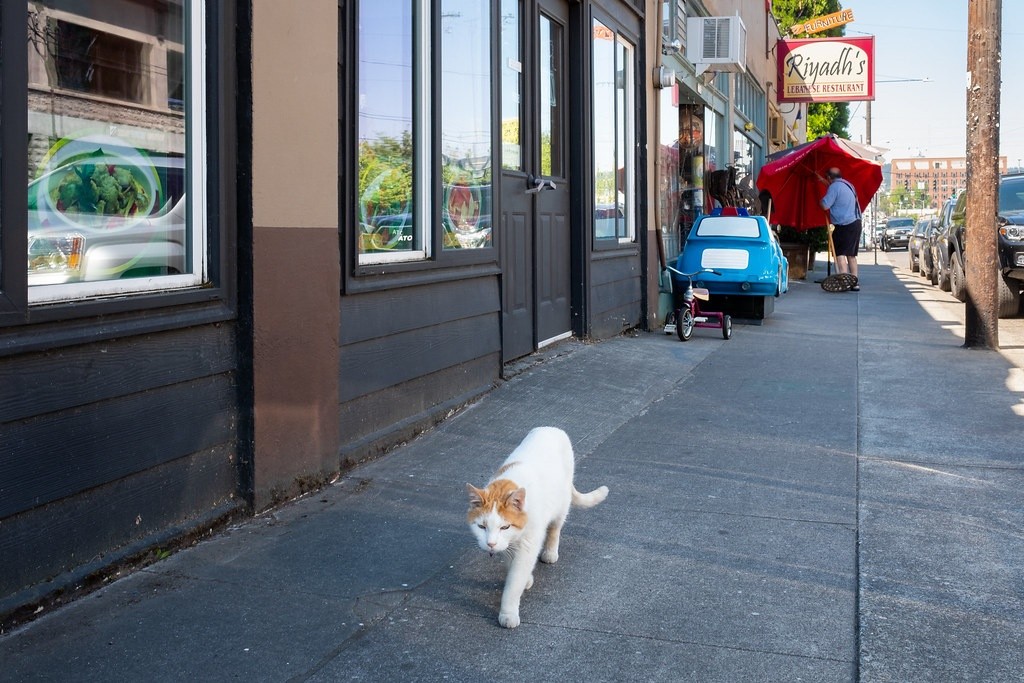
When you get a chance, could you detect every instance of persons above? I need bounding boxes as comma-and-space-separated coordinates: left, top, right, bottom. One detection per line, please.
819, 167, 863, 291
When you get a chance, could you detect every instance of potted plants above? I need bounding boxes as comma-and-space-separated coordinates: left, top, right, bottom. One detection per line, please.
774, 225, 830, 279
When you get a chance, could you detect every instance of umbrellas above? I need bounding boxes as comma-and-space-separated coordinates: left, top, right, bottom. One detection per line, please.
765, 134, 890, 164
756, 137, 883, 231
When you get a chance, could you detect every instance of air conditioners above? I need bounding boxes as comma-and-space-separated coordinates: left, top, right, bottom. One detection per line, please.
686, 15, 747, 73
769, 117, 786, 142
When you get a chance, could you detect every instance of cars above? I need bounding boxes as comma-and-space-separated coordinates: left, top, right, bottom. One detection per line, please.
27, 127, 626, 308
907, 216, 931, 272
673, 206, 789, 318
868, 218, 888, 247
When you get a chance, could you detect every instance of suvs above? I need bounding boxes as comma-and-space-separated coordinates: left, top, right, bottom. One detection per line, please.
881, 217, 917, 252
918, 172, 1024, 318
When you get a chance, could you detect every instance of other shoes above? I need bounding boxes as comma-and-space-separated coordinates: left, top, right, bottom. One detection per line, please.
849, 282, 860, 291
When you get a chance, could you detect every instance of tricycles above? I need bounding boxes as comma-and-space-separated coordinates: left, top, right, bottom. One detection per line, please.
663, 264, 733, 342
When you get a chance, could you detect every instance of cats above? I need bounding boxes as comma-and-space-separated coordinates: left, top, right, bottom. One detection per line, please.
465, 425, 610, 630
